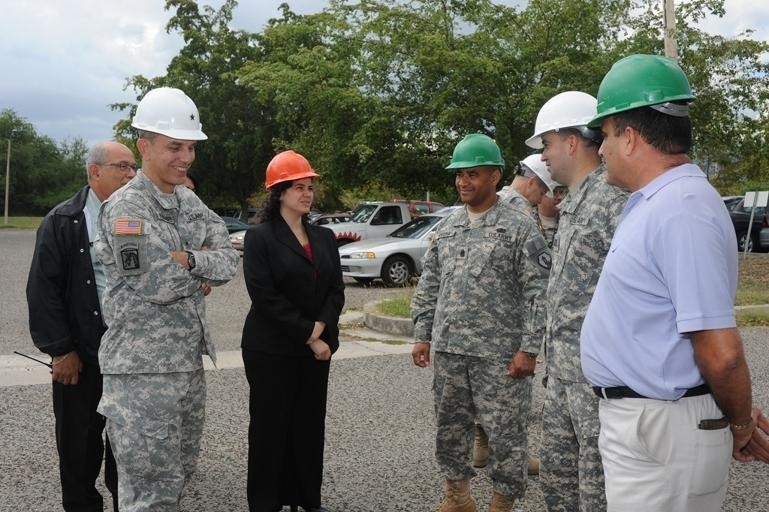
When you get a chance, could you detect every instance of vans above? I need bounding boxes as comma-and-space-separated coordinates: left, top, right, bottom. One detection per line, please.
393, 199, 445, 219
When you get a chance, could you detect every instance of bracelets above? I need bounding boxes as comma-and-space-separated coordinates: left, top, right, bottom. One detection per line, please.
51, 353, 70, 366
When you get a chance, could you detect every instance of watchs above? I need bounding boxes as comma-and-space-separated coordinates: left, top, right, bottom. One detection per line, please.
186, 250, 196, 272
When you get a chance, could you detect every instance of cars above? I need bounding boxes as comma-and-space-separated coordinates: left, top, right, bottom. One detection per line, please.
214, 208, 263, 256
339, 213, 447, 287
722, 195, 769, 253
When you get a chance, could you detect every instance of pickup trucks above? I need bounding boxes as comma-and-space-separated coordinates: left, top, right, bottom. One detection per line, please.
320, 203, 411, 247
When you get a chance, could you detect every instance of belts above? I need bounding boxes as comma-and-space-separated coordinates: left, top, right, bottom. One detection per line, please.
594, 385, 711, 400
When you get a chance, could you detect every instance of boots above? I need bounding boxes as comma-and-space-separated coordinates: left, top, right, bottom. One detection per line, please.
485, 491, 514, 512
526, 455, 539, 475
436, 477, 476, 511
473, 441, 493, 468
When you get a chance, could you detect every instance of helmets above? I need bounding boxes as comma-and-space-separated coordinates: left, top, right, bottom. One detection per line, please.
587, 53, 698, 129
524, 90, 598, 149
265, 151, 321, 190
131, 87, 208, 141
445, 133, 506, 171
519, 151, 564, 200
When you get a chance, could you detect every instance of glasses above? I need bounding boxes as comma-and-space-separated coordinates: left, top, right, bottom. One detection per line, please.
95, 162, 136, 171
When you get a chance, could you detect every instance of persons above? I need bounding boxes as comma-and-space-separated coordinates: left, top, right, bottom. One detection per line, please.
25, 140, 137, 512
93, 87, 239, 512
240, 151, 345, 511
410, 53, 769, 511
